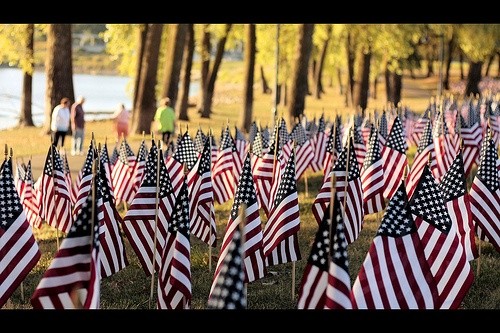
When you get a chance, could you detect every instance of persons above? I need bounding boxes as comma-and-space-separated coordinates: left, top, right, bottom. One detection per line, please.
70, 97, 85, 156
154, 97, 175, 145
51, 97, 70, 148
113, 104, 130, 143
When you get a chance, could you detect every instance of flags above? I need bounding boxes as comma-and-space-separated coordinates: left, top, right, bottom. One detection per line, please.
30, 174, 95, 309
0, 89, 500, 310
351, 181, 440, 309
156, 176, 193, 309
